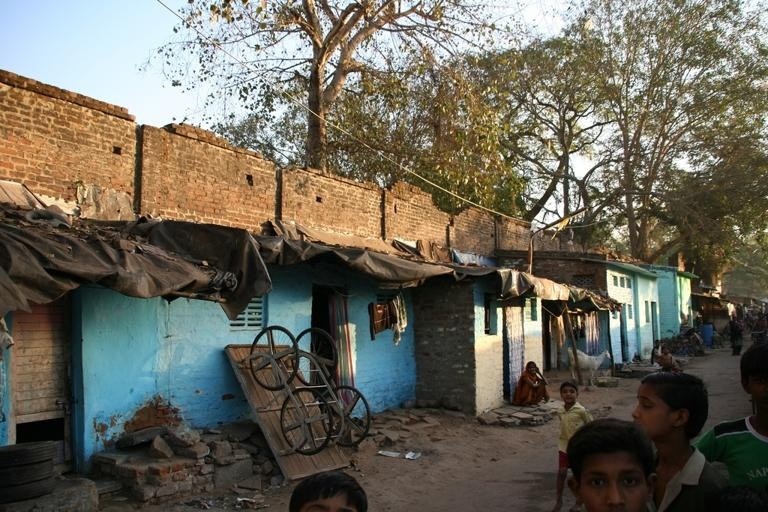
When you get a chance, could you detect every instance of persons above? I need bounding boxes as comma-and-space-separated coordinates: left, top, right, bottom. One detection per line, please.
287, 469, 367, 511
628, 370, 732, 511
555, 381, 593, 510
566, 417, 659, 512
691, 338, 768, 511
674, 485, 768, 512
730, 309, 742, 356
650, 339, 663, 364
510, 361, 552, 407
662, 346, 683, 374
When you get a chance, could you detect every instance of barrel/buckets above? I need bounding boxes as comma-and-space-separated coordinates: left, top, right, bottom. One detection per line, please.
702, 321, 714, 345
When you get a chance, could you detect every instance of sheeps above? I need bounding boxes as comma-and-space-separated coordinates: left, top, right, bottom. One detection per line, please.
568, 347, 611, 380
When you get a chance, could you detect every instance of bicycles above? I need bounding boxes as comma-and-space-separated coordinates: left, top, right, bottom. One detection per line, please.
660, 327, 699, 358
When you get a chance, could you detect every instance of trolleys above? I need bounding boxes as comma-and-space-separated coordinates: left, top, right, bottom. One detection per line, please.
223, 323, 374, 480
